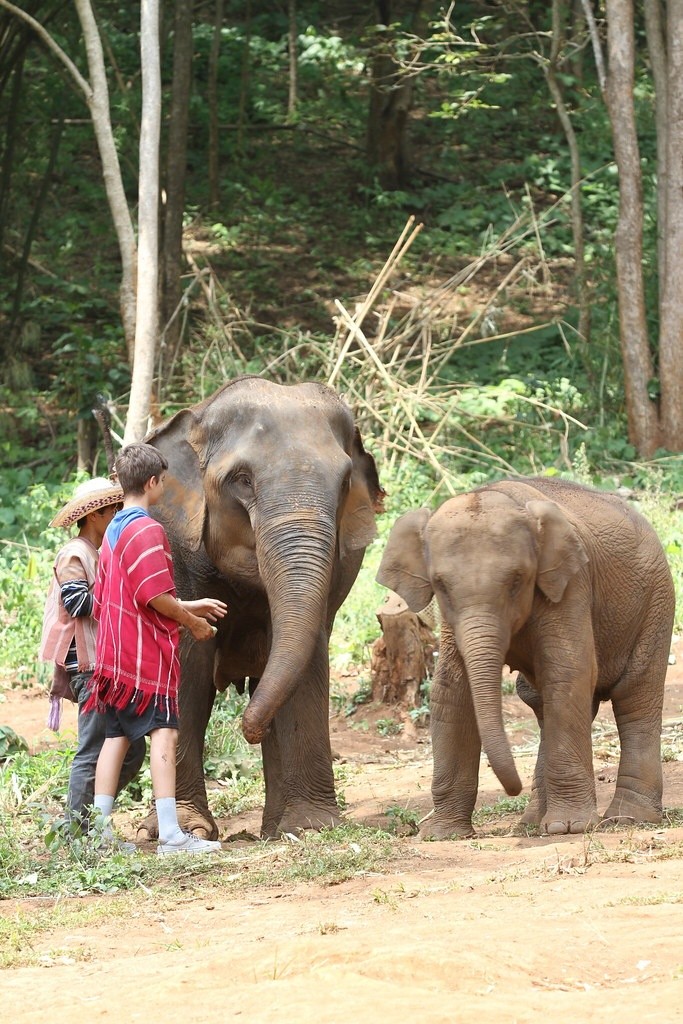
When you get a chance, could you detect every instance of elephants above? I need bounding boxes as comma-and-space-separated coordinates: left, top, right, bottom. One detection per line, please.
374, 477, 677, 843
136, 375, 388, 846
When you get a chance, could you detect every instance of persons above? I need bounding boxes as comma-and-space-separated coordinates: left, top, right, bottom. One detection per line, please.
42, 477, 147, 844
90, 442, 229, 858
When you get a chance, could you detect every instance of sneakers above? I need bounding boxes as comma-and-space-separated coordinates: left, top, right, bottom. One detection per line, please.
156, 831, 221, 857
88, 831, 136, 855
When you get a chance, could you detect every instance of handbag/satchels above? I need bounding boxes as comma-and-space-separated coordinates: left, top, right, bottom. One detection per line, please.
47, 664, 79, 732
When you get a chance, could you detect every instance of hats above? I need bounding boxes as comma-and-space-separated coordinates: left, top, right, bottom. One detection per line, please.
48, 476, 124, 528
